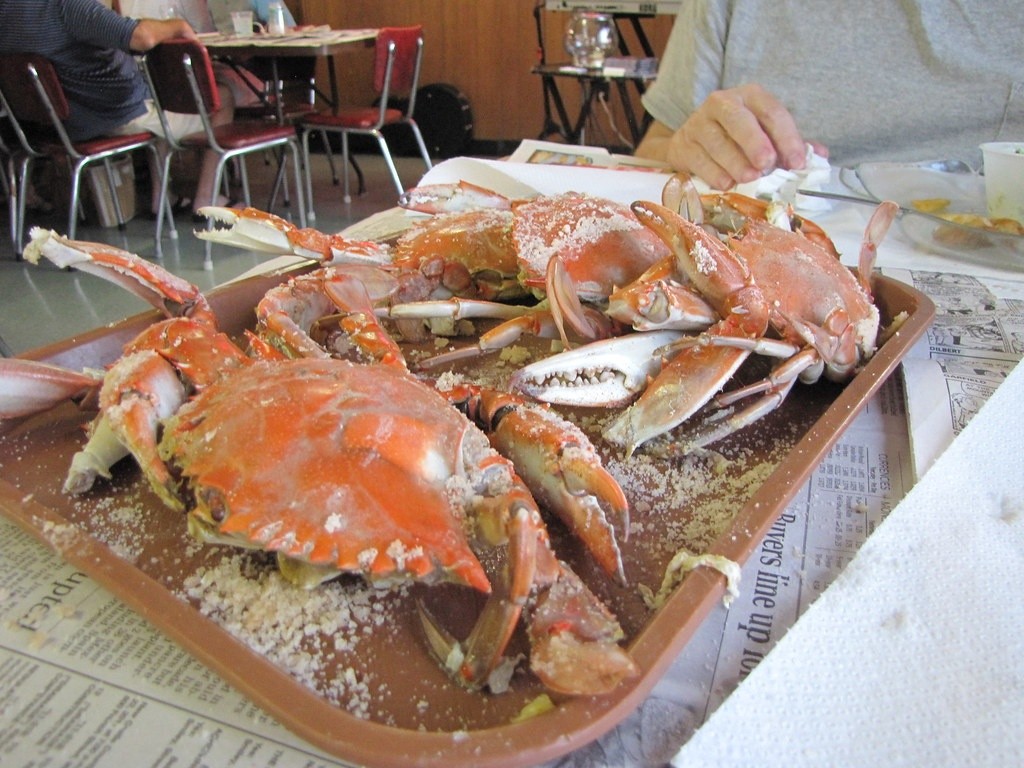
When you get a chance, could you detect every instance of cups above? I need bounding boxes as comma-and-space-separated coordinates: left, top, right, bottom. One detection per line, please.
230, 11, 253, 39
980, 142, 1024, 228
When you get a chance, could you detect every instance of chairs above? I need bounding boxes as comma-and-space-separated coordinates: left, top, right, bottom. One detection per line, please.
142, 39, 307, 271
0, 52, 179, 263
302, 24, 433, 221
232, 57, 339, 206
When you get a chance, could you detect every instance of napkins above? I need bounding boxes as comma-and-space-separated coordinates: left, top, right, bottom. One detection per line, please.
668, 356, 1024, 768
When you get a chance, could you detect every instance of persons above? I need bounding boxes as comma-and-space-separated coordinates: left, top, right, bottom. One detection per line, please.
631, 0, 1023, 191
0, 0, 246, 226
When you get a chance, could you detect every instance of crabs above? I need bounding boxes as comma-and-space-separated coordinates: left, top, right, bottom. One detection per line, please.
22, 177, 899, 696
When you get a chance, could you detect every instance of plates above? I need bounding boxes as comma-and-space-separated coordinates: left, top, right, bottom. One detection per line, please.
1, 226, 936, 768
839, 159, 1024, 273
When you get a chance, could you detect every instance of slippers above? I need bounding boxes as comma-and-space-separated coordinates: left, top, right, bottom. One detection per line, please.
193, 200, 236, 221
152, 195, 192, 218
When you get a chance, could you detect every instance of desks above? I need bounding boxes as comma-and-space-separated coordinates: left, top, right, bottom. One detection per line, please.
195, 28, 380, 213
532, 57, 659, 149
0, 140, 1024, 768
